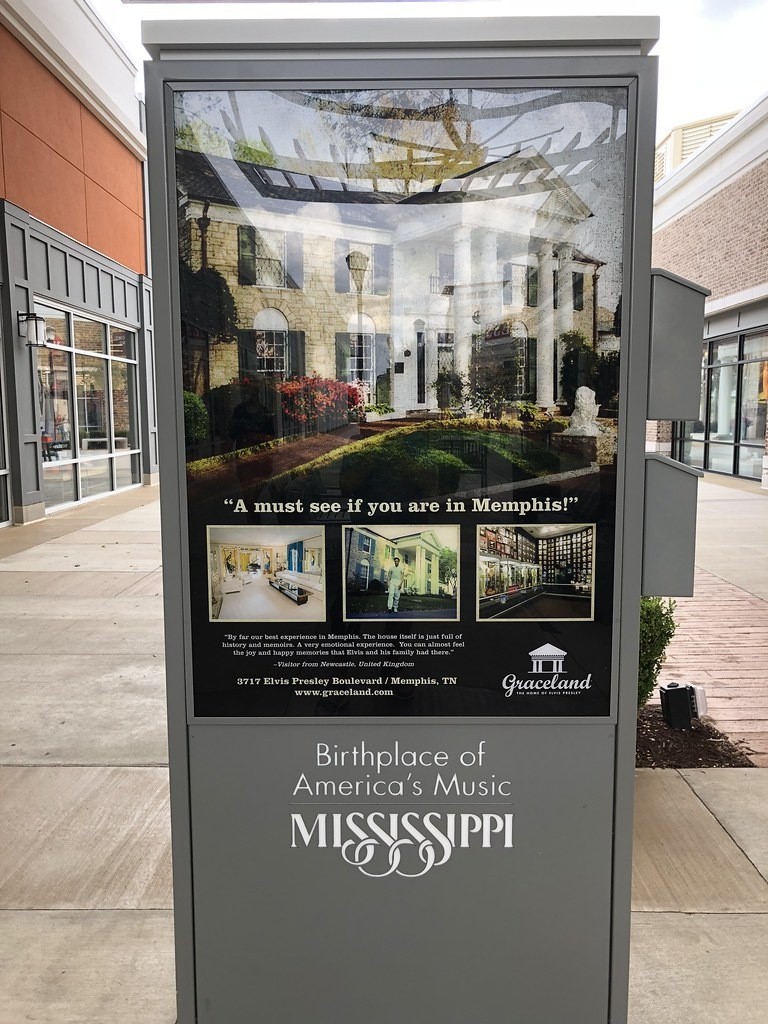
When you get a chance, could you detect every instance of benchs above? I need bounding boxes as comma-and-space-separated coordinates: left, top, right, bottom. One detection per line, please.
81, 435, 131, 451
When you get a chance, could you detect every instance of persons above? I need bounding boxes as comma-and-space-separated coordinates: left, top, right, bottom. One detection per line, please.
387, 558, 404, 613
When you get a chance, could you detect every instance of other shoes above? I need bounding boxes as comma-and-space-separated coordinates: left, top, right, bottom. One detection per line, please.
388, 607, 392, 613
393, 608, 398, 613
